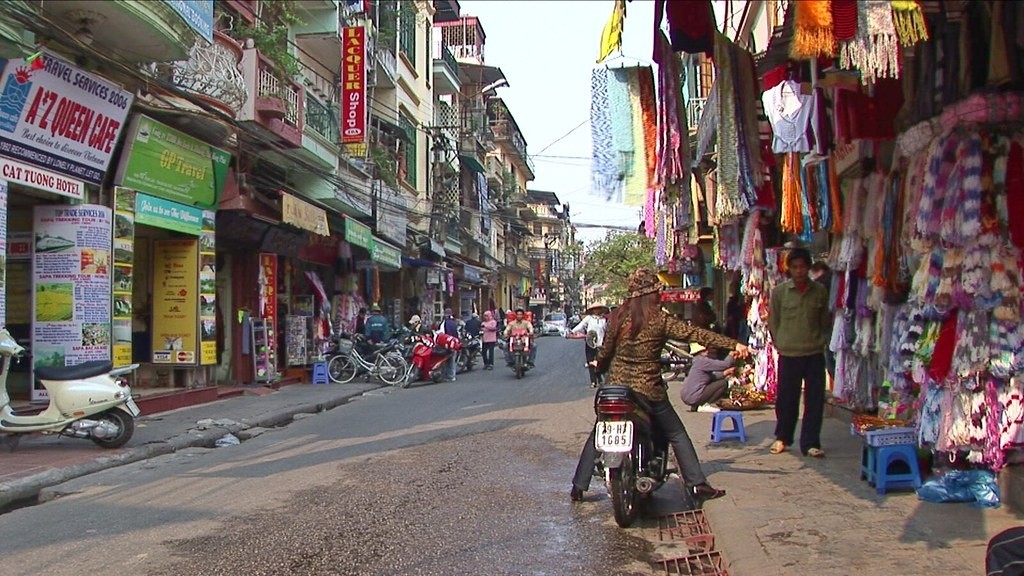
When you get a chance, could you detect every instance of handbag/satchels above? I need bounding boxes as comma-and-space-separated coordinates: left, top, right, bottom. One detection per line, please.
916, 470, 1001, 506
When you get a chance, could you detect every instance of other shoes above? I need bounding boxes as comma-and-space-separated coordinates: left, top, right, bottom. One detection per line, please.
507, 362, 514, 367
482, 364, 488, 369
591, 382, 596, 388
487, 364, 493, 370
570, 486, 583, 500
691, 483, 726, 500
451, 376, 456, 381
527, 362, 534, 368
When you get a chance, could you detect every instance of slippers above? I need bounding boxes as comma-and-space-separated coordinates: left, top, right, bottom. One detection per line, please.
807, 447, 823, 457
769, 439, 786, 454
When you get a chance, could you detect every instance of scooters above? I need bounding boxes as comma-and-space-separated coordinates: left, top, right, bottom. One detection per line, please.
0, 326, 142, 450
318, 325, 482, 385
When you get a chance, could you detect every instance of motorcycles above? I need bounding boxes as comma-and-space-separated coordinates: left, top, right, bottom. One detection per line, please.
501, 329, 535, 379
402, 330, 462, 389
585, 346, 689, 529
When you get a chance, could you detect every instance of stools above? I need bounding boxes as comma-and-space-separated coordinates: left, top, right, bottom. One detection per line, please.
711, 411, 746, 443
860, 441, 923, 495
313, 361, 329, 384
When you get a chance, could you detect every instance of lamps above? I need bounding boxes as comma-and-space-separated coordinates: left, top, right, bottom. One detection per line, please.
75, 18, 94, 46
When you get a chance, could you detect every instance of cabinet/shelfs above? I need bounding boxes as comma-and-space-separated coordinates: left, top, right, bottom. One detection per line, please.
247, 317, 278, 383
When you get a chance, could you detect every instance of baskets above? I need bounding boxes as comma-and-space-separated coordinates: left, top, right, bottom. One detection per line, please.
719, 397, 765, 410
851, 426, 916, 446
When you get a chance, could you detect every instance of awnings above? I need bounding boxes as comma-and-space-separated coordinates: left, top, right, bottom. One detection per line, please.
251, 170, 454, 274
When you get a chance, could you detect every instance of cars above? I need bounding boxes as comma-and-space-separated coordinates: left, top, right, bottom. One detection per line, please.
539, 311, 568, 336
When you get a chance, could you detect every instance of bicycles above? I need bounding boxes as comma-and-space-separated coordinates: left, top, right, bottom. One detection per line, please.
327, 334, 408, 388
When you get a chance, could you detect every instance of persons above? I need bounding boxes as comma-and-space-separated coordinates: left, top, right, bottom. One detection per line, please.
346, 306, 538, 382
570, 269, 760, 501
569, 302, 610, 388
680, 281, 745, 413
767, 247, 829, 456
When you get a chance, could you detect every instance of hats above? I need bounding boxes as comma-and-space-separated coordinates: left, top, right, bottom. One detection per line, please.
472, 313, 479, 318
626, 267, 663, 298
409, 314, 420, 324
370, 306, 381, 311
586, 302, 609, 316
689, 342, 706, 354
